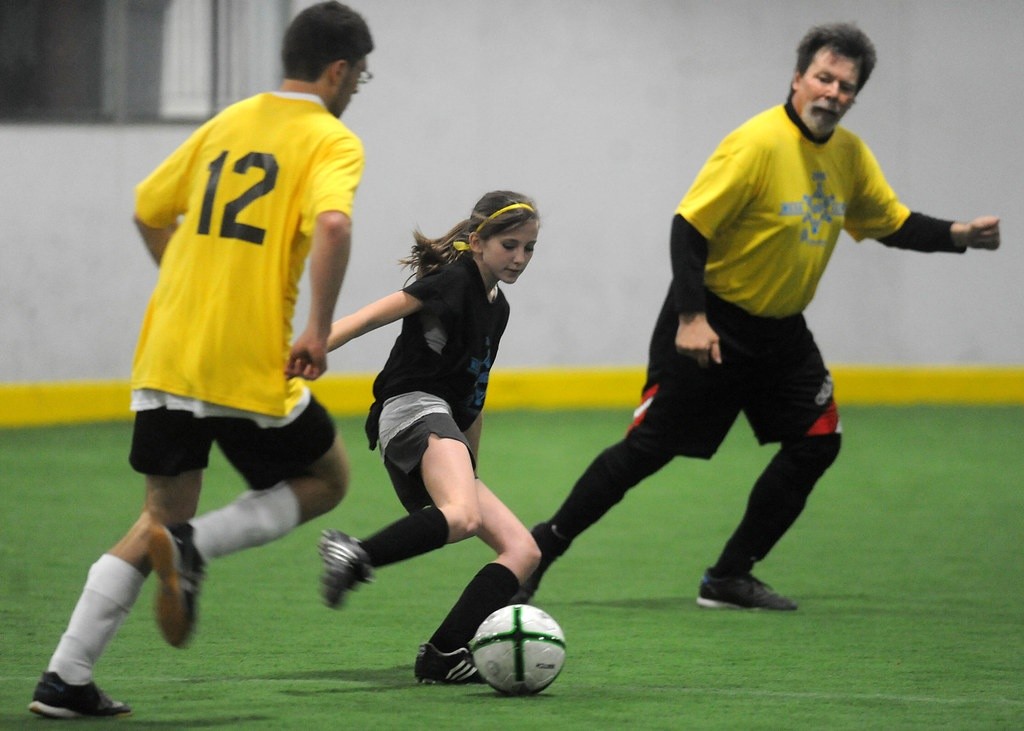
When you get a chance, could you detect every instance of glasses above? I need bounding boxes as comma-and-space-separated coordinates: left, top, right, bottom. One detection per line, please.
344, 58, 373, 84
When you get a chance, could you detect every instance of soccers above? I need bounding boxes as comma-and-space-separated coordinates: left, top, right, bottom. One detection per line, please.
467, 603, 566, 692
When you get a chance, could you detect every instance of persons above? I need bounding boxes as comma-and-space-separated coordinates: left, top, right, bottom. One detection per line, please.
315, 189, 545, 685
498, 23, 1003, 612
24, 0, 377, 723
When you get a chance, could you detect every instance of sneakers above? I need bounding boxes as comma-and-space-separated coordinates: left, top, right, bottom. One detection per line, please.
509, 520, 570, 610
28, 671, 132, 717
413, 641, 487, 686
316, 529, 373, 610
149, 521, 207, 649
695, 565, 798, 612
